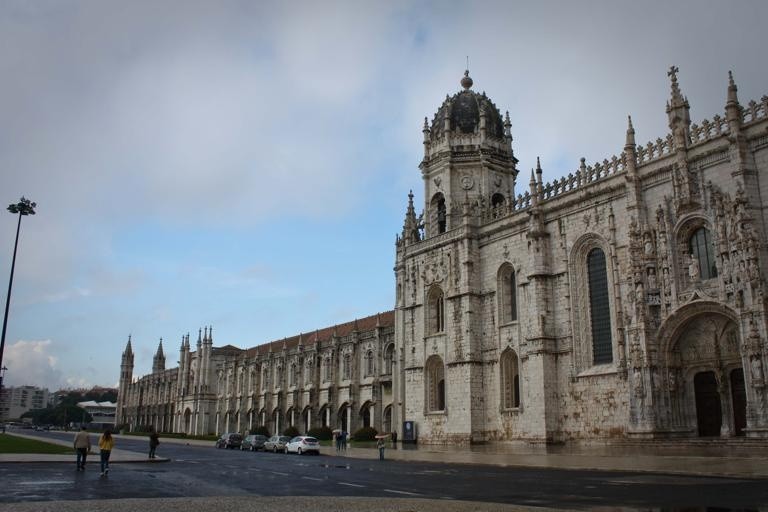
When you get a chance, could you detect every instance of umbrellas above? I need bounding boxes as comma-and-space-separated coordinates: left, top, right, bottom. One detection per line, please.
375, 431, 391, 439
332, 428, 343, 434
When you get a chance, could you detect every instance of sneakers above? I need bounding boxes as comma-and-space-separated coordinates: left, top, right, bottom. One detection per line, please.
100, 468, 109, 476
76, 466, 85, 471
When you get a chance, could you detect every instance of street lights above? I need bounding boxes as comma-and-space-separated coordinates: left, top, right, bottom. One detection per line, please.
0, 195, 36, 368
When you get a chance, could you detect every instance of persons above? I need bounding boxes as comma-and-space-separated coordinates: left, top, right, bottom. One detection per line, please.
74, 425, 91, 471
98, 428, 113, 476
149, 431, 159, 458
642, 180, 760, 297
336, 433, 341, 451
752, 356, 762, 379
714, 367, 725, 384
340, 432, 347, 451
633, 368, 643, 389
377, 437, 385, 461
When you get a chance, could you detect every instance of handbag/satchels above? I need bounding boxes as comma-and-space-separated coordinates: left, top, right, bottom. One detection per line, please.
156, 439, 159, 445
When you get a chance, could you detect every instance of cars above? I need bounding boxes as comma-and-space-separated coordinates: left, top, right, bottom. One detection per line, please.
216, 433, 320, 455
